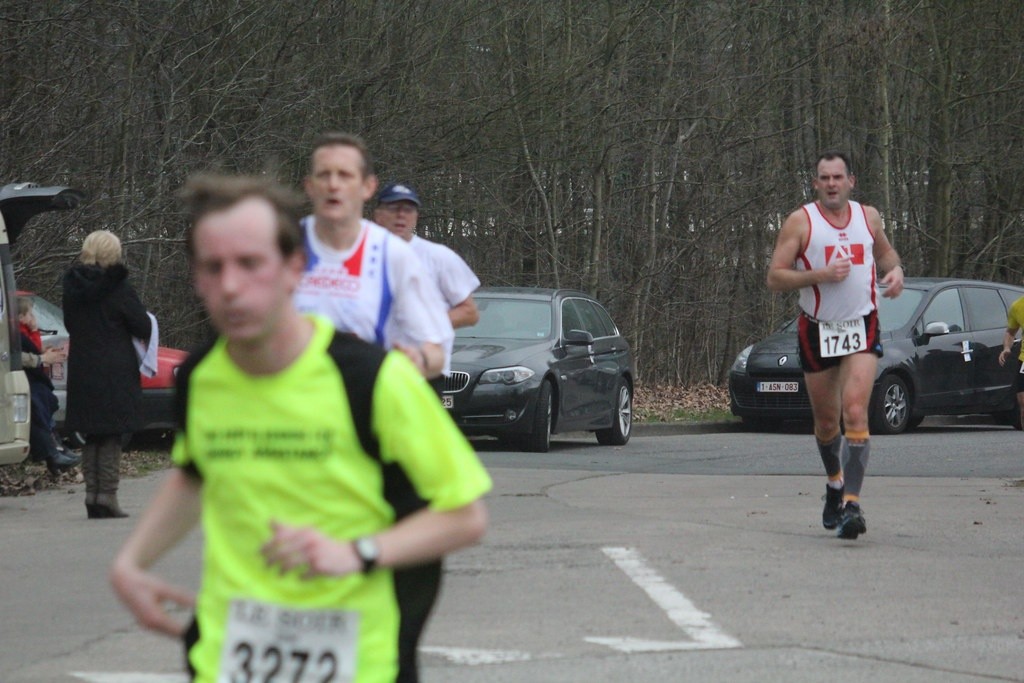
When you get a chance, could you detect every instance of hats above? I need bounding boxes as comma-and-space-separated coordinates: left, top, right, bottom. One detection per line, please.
378, 182, 420, 204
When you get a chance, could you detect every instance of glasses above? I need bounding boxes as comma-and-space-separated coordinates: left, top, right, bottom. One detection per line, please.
378, 204, 417, 214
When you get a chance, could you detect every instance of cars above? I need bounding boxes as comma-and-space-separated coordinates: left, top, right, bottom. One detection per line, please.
429, 287, 637, 454
13, 287, 190, 446
728, 275, 1024, 435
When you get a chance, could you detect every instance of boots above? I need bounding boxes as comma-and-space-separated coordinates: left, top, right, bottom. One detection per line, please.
96, 433, 129, 517
82, 434, 98, 517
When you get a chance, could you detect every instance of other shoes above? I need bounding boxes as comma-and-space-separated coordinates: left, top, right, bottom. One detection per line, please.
46, 452, 83, 476
61, 446, 82, 460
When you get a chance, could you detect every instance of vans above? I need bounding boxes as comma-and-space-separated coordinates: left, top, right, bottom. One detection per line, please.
0, 182, 88, 470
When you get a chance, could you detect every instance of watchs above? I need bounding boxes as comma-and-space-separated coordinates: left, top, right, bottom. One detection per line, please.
353, 536, 379, 572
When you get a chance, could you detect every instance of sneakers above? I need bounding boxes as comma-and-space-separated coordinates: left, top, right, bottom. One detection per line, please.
822, 483, 844, 528
836, 500, 866, 540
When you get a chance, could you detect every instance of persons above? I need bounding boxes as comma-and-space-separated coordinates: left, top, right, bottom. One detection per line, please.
288, 132, 479, 405
109, 176, 487, 683
18, 292, 84, 474
61, 230, 157, 518
766, 151, 904, 541
1000, 293, 1024, 429
